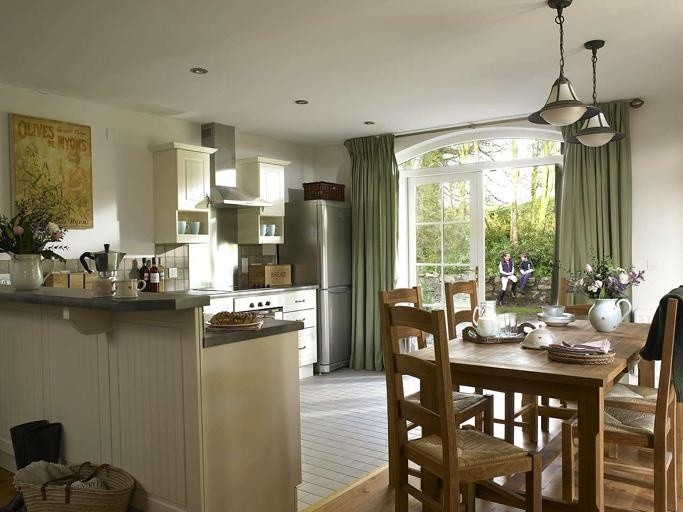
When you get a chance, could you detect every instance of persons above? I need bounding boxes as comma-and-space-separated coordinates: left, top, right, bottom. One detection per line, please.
517, 251, 536, 299
496, 254, 517, 305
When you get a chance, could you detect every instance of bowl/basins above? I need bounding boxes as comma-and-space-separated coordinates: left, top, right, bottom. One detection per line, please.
541, 304, 565, 316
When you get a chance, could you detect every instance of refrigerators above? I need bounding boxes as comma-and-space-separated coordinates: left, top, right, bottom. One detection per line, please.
278, 199, 352, 376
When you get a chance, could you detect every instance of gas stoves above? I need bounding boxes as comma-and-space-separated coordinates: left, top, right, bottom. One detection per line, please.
219, 284, 275, 293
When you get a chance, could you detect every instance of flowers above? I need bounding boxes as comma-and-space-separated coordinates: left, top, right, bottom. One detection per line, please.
0, 197, 67, 258
549, 245, 647, 298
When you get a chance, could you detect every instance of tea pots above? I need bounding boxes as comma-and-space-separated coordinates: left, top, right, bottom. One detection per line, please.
472, 300, 500, 338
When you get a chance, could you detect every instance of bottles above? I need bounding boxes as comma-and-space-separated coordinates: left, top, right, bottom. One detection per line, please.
149, 258, 159, 290
159, 257, 166, 290
140, 258, 148, 291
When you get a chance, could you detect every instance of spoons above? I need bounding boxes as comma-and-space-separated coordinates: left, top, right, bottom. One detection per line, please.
562, 340, 603, 350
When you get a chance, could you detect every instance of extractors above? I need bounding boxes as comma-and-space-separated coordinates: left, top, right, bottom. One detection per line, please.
201, 122, 264, 208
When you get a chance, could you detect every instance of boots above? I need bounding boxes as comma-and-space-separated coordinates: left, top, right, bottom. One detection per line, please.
510, 282, 518, 299
498, 290, 506, 307
0, 418, 63, 512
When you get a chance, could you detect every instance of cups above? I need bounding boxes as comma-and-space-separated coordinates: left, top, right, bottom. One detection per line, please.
507, 313, 518, 334
114, 279, 146, 296
189, 222, 200, 234
260, 224, 269, 236
267, 224, 278, 236
497, 312, 510, 337
178, 221, 189, 234
91, 276, 117, 295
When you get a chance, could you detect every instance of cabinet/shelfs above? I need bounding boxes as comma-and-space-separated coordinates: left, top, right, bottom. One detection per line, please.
183, 276, 321, 382
235, 151, 293, 246
147, 141, 229, 242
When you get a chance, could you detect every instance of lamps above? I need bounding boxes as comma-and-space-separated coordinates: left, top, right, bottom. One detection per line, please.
563, 37, 627, 149
528, 0, 602, 129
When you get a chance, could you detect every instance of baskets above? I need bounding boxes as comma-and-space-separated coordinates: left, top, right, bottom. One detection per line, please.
20, 460, 137, 511
303, 180, 345, 202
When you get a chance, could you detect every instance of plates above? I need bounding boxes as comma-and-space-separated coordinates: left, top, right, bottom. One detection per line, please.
90, 294, 114, 298
537, 314, 576, 327
204, 320, 264, 327
112, 295, 139, 299
520, 342, 550, 350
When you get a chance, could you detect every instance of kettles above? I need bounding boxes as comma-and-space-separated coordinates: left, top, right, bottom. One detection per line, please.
80, 244, 126, 277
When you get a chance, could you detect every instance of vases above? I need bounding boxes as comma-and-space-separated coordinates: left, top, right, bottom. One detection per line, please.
3, 252, 56, 292
586, 293, 633, 336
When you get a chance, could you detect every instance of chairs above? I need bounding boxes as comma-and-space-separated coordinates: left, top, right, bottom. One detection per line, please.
373, 303, 544, 512
560, 284, 683, 512
540, 276, 624, 437
441, 278, 535, 449
376, 283, 495, 490
